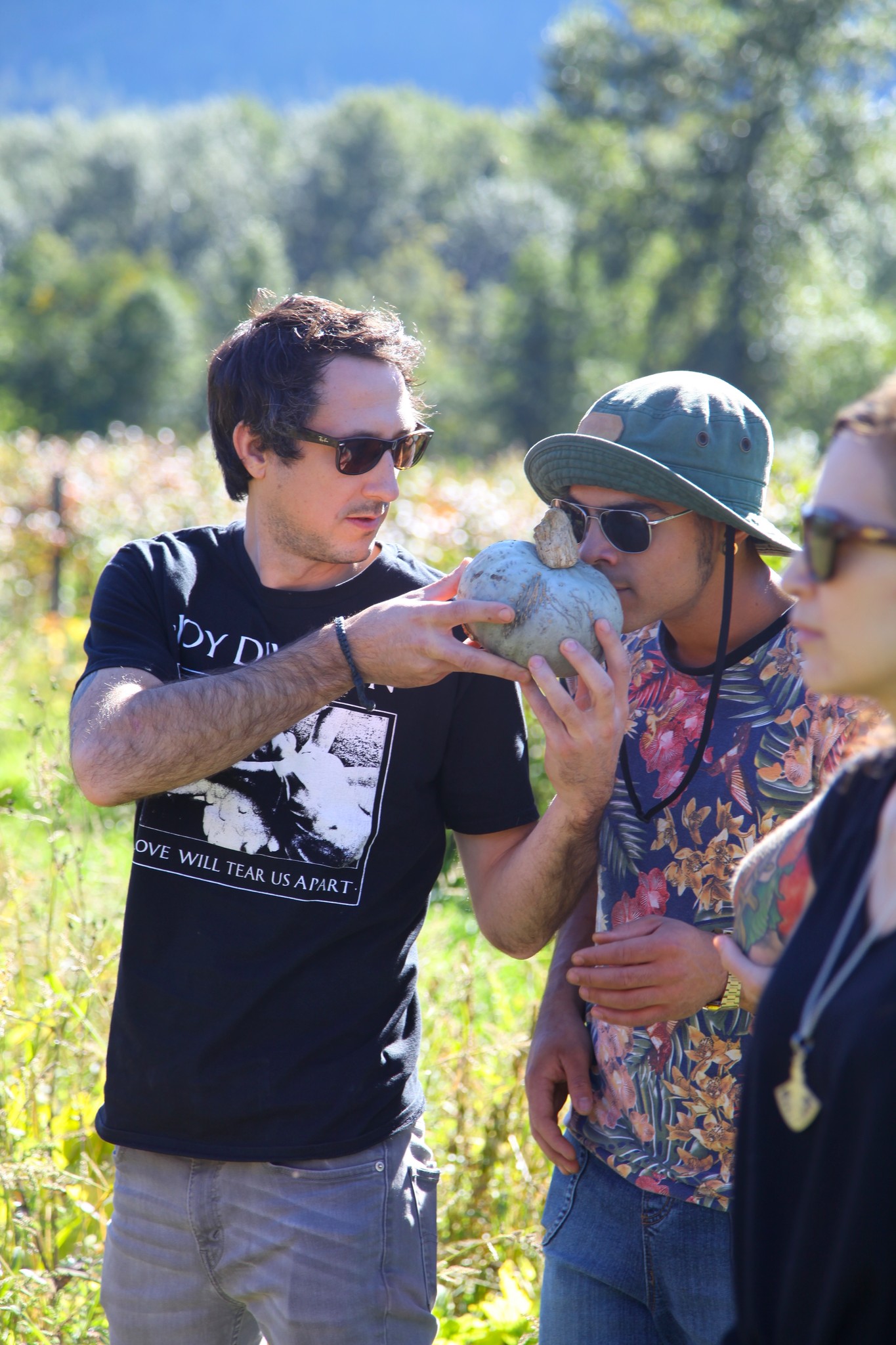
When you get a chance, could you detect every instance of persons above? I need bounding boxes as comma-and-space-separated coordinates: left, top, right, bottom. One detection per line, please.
722, 375, 895, 1344
519, 368, 884, 1345
67, 291, 633, 1345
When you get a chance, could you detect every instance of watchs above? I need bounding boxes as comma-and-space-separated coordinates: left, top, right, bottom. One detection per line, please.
701, 975, 743, 1011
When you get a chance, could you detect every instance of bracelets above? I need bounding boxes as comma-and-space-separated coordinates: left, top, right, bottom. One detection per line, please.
333, 616, 376, 712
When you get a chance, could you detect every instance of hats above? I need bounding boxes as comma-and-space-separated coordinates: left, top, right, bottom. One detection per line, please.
530, 370, 805, 557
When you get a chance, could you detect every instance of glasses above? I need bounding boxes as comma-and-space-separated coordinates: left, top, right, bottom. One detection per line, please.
291, 421, 432, 473
547, 495, 697, 552
797, 503, 896, 584
